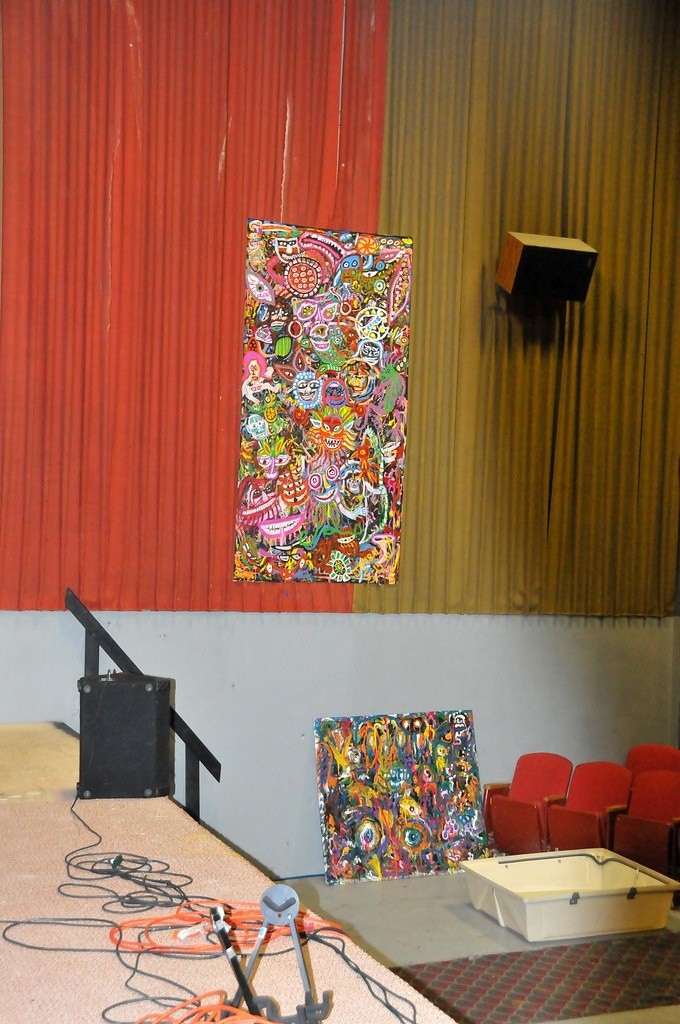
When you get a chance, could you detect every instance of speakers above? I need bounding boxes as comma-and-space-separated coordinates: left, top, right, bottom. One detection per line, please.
74, 669, 177, 799
494, 230, 599, 302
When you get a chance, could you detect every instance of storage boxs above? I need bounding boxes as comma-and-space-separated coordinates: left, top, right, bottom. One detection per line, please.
459, 848, 680, 942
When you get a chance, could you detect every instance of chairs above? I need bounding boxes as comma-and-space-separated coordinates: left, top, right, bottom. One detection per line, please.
481, 745, 679, 909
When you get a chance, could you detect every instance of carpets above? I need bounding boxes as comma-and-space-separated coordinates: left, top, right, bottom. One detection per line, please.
389, 934, 680, 1024
